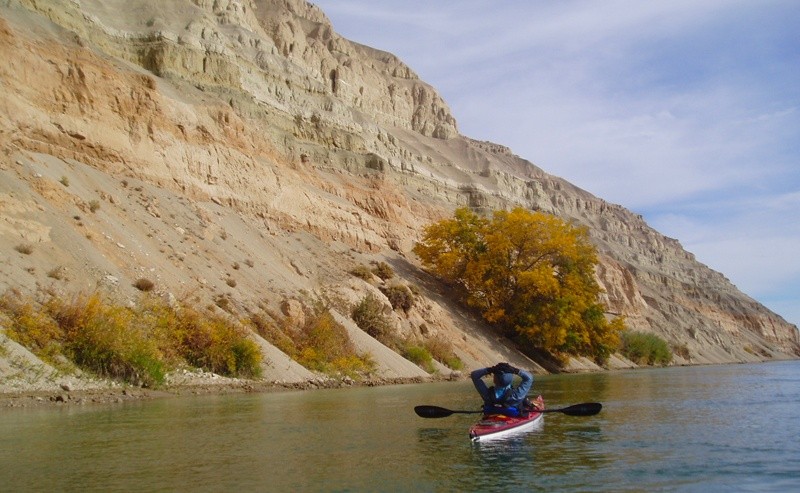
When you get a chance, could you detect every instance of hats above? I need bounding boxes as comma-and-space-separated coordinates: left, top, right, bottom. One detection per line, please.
493, 372, 512, 386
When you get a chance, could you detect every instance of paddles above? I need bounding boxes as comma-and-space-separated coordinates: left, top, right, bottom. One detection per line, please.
414, 400, 603, 419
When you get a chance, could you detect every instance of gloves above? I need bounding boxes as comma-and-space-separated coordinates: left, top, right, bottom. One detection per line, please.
487, 363, 519, 376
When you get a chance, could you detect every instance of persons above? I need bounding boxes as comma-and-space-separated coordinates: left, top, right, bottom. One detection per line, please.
471, 362, 534, 412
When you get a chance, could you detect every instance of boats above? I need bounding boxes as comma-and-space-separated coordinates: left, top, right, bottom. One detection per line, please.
468, 394, 546, 445
